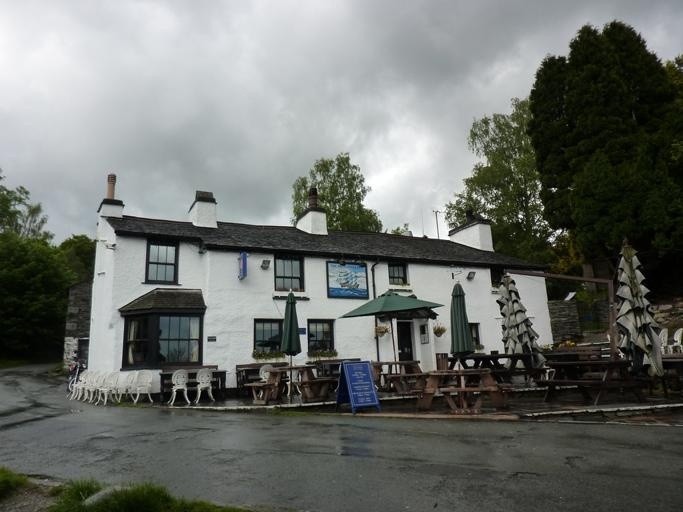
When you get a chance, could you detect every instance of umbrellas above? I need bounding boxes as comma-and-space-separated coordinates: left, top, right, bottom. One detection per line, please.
496, 272, 555, 385
338, 290, 445, 375
280, 289, 302, 404
614, 236, 665, 378
450, 284, 475, 370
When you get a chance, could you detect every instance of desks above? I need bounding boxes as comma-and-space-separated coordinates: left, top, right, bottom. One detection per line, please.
159, 372, 226, 405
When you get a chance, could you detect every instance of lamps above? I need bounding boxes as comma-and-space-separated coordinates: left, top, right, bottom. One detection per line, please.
467, 271, 476, 280
67, 368, 216, 406
434, 323, 447, 337
261, 259, 270, 270
375, 325, 390, 337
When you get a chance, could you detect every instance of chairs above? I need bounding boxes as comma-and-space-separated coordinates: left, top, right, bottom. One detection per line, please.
606, 327, 683, 358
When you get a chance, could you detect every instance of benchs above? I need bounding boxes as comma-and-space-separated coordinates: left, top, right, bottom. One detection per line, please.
243, 354, 682, 414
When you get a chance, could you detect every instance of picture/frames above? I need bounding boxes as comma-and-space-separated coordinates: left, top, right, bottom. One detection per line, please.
326, 260, 369, 299
